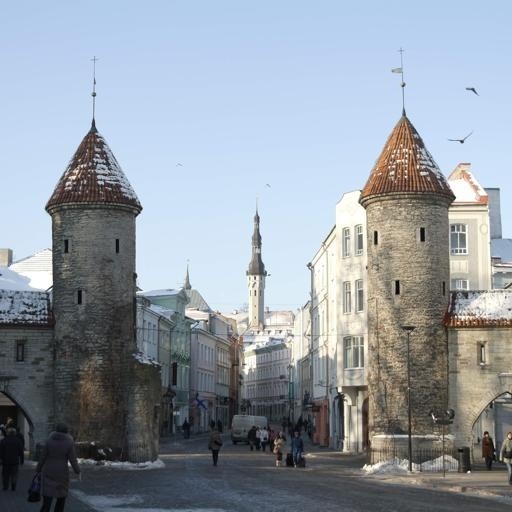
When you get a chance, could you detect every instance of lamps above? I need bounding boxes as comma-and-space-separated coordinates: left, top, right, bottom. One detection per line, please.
172, 406, 180, 417
336, 386, 348, 403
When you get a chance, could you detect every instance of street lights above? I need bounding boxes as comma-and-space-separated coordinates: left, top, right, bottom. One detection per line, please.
400, 325, 417, 472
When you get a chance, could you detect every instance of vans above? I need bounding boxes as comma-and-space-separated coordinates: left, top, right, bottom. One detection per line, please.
231, 415, 268, 445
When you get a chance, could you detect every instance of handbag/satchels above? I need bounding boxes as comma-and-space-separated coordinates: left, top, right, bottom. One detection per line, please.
273, 447, 279, 454
299, 457, 305, 467
286, 453, 294, 466
27, 470, 41, 502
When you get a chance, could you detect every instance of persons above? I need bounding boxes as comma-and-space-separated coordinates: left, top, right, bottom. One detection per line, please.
207, 427, 221, 466
481, 430, 496, 470
0, 426, 25, 492
499, 431, 512, 485
210, 417, 215, 432
248, 412, 309, 469
15, 426, 25, 465
217, 419, 222, 433
182, 419, 191, 438
34, 421, 85, 512
